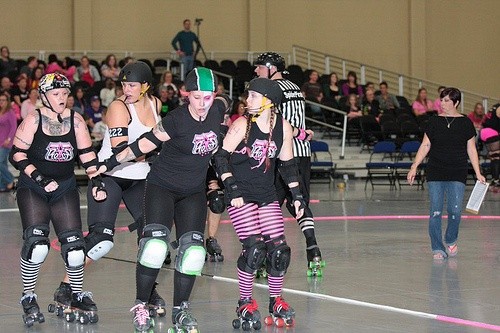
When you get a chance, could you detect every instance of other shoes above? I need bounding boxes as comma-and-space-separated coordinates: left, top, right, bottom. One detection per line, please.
0, 182, 15, 193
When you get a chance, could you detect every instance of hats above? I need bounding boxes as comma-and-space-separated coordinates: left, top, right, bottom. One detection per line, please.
90, 95, 99, 103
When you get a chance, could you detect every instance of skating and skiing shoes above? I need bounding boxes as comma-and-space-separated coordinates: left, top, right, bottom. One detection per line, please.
130, 299, 155, 333
253, 255, 269, 278
48, 281, 73, 317
65, 290, 99, 325
20, 291, 44, 328
205, 236, 224, 262
232, 297, 261, 331
167, 300, 201, 333
306, 247, 325, 277
489, 178, 500, 192
264, 296, 295, 328
148, 282, 167, 317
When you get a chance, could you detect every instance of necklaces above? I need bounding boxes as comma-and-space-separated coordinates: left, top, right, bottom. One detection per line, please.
445, 116, 455, 128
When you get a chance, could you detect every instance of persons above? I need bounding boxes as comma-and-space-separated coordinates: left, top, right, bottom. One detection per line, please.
8, 73, 107, 327
215, 78, 306, 330
87, 67, 225, 333
407, 88, 485, 261
254, 52, 325, 279
0, 19, 500, 262
48, 61, 167, 317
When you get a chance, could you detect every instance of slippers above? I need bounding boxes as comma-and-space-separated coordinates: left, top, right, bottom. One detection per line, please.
432, 253, 445, 260
447, 243, 458, 256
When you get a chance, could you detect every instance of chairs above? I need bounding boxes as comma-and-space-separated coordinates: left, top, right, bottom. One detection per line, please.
286, 65, 494, 190
15, 59, 258, 97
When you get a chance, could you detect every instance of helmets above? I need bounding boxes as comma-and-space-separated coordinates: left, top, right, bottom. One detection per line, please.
38, 73, 71, 94
118, 60, 152, 83
245, 78, 284, 106
184, 66, 218, 92
253, 51, 285, 72
